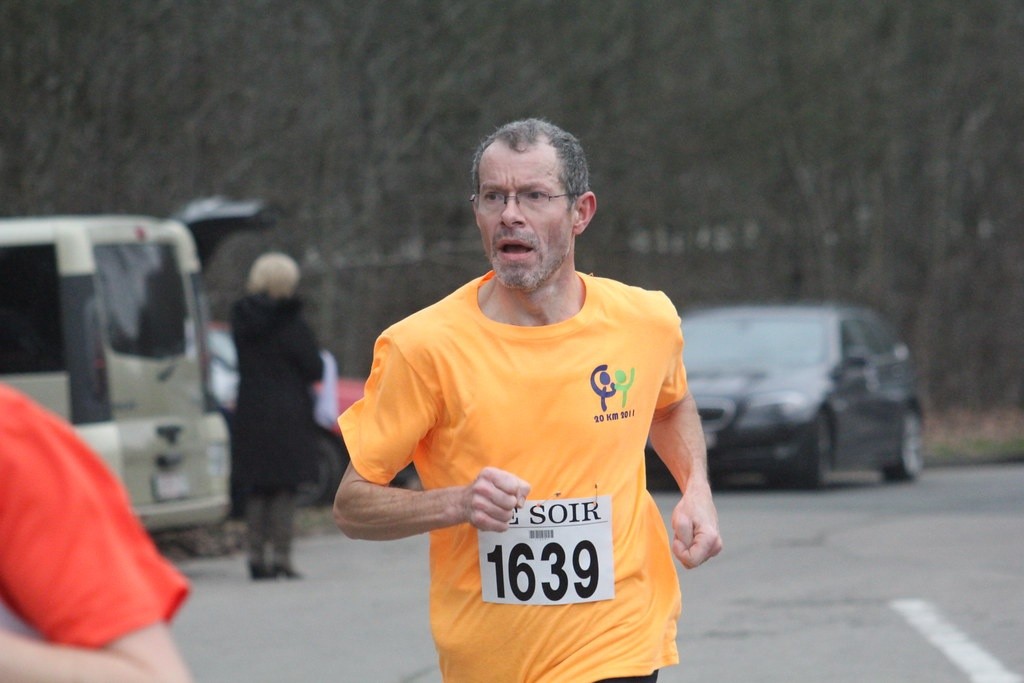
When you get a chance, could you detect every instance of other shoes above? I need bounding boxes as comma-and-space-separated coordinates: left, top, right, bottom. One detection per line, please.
251, 564, 295, 579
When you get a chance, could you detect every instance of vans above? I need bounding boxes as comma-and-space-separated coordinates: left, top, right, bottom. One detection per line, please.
0, 194, 274, 562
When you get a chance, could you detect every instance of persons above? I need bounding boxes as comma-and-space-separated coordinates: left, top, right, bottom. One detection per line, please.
332, 119, 723, 683
0, 383, 192, 683
206, 250, 323, 579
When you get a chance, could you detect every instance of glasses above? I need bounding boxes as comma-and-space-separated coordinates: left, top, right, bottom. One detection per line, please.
470, 191, 577, 218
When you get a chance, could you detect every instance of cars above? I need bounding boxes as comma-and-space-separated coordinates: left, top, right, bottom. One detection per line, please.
642, 304, 923, 492
